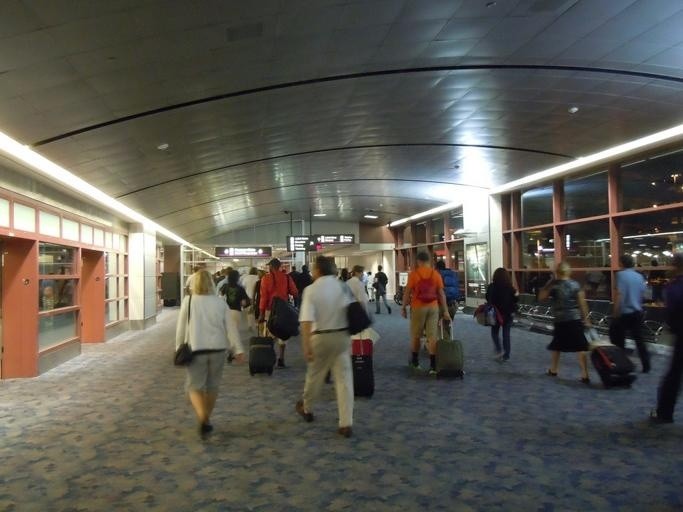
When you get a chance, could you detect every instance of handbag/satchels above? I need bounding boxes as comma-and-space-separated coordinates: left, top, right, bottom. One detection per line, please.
349, 302, 371, 335
476, 303, 496, 326
174, 345, 193, 366
267, 296, 300, 341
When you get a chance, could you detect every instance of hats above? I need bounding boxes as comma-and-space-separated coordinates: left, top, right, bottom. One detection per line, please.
266, 258, 280, 265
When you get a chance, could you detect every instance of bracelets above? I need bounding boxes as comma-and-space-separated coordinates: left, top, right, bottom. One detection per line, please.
402, 308, 405, 310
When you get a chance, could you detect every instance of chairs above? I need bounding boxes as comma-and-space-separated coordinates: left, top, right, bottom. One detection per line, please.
512, 295, 664, 355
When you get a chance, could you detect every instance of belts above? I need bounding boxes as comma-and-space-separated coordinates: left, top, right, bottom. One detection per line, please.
312, 327, 349, 335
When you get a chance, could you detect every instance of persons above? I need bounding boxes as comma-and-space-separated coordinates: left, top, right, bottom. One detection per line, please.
537, 263, 594, 382
649, 261, 682, 430
610, 254, 652, 373
434, 261, 459, 342
484, 268, 517, 362
183, 254, 392, 385
174, 268, 246, 441
584, 259, 666, 302
294, 256, 359, 438
399, 251, 452, 376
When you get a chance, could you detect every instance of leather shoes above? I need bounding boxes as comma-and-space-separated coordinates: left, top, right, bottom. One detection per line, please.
296, 401, 313, 422
339, 427, 351, 436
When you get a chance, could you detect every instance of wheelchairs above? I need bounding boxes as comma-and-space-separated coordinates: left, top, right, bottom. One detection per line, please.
394, 287, 411, 306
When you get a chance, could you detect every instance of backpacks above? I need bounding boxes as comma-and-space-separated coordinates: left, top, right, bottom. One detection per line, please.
416, 269, 437, 302
439, 270, 459, 300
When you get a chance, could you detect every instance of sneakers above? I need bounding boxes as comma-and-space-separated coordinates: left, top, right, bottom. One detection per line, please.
278, 359, 285, 368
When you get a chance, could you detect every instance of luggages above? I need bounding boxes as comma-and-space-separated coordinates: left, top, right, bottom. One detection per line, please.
587, 326, 637, 388
436, 317, 465, 380
351, 332, 374, 398
249, 320, 273, 377
